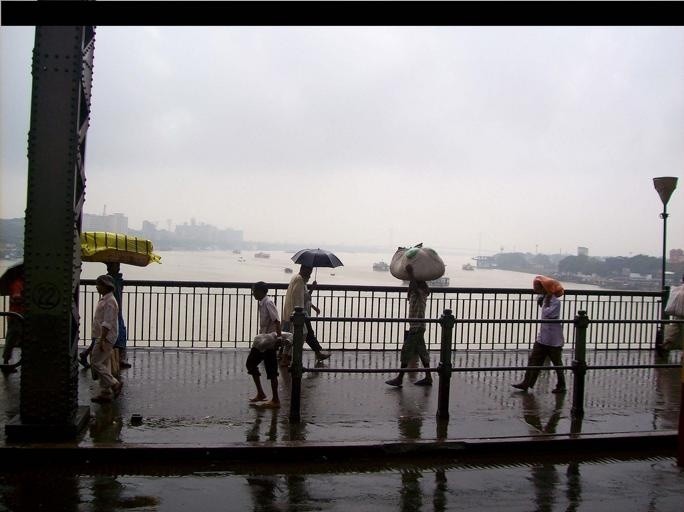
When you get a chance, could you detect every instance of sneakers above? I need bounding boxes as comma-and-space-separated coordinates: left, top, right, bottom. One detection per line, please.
112, 380, 124, 399
551, 387, 566, 394
318, 353, 331, 361
90, 391, 112, 403
510, 383, 529, 392
120, 360, 132, 368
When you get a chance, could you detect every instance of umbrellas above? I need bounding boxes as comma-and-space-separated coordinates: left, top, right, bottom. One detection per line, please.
290, 247, 345, 281
0, 261, 24, 297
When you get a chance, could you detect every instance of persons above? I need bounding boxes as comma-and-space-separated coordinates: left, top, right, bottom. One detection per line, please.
0, 274, 22, 372
245, 280, 281, 408
382, 264, 434, 388
302, 273, 331, 362
88, 274, 124, 403
77, 260, 131, 370
280, 265, 312, 372
510, 275, 567, 392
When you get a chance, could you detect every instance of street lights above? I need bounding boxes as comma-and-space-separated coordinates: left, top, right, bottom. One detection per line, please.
652, 176, 679, 290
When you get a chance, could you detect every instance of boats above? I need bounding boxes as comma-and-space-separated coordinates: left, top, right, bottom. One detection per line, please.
426, 277, 450, 287
232, 249, 241, 254
255, 252, 271, 258
374, 260, 390, 272
462, 264, 475, 270
285, 268, 293, 273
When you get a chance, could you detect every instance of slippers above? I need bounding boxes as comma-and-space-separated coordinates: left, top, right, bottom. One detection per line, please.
414, 378, 434, 386
260, 402, 282, 409
77, 355, 90, 368
384, 377, 404, 388
249, 395, 269, 402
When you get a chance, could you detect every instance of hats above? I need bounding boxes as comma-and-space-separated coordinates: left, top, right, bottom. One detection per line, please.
251, 281, 269, 293
96, 274, 116, 289
